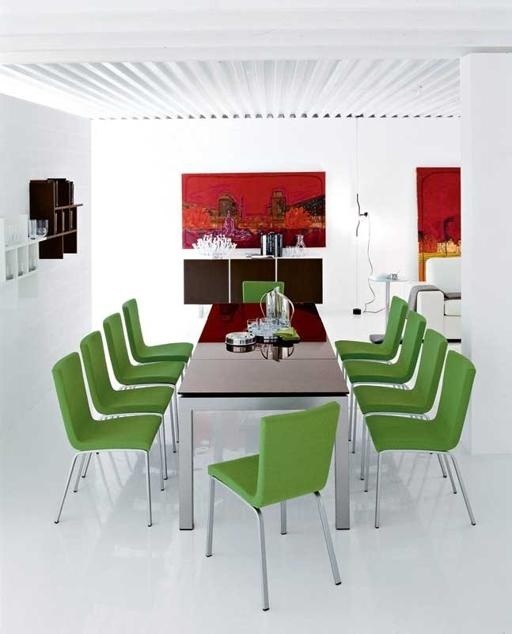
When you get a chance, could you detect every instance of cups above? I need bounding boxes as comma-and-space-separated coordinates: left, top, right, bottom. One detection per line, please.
3, 218, 49, 281
247, 318, 290, 339
286, 245, 294, 257
436, 242, 445, 252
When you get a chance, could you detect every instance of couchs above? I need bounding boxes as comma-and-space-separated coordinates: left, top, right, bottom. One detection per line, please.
416, 257, 462, 342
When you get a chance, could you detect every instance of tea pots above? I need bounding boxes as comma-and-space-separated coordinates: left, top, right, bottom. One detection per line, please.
258, 286, 295, 322
444, 238, 457, 253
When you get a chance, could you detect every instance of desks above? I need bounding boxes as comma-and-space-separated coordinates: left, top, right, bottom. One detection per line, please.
177, 303, 353, 531
370, 271, 409, 344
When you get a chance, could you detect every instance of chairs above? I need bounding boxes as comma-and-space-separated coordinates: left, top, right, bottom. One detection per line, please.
334, 295, 478, 529
242, 280, 285, 303
205, 401, 344, 612
52, 299, 193, 527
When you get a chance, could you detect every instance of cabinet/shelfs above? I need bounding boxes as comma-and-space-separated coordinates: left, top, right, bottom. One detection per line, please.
2, 176, 84, 283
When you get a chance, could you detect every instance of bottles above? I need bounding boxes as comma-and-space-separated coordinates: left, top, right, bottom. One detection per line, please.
295, 234, 307, 256
